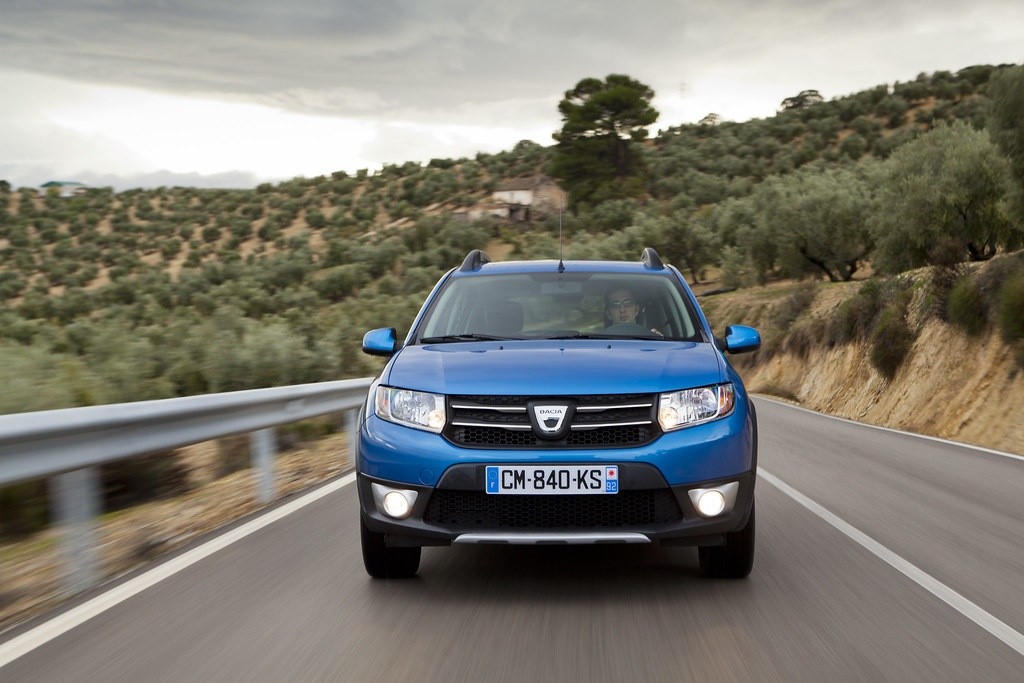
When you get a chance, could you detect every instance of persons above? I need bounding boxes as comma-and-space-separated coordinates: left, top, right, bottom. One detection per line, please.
604, 283, 664, 337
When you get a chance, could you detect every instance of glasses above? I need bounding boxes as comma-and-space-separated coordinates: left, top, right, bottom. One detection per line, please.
608, 299, 635, 309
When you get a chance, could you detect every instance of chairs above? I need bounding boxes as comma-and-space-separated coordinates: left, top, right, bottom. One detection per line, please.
483, 301, 526, 337
602, 306, 643, 327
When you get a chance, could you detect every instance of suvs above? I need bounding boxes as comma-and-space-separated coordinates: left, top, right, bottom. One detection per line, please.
355, 248, 764, 582
